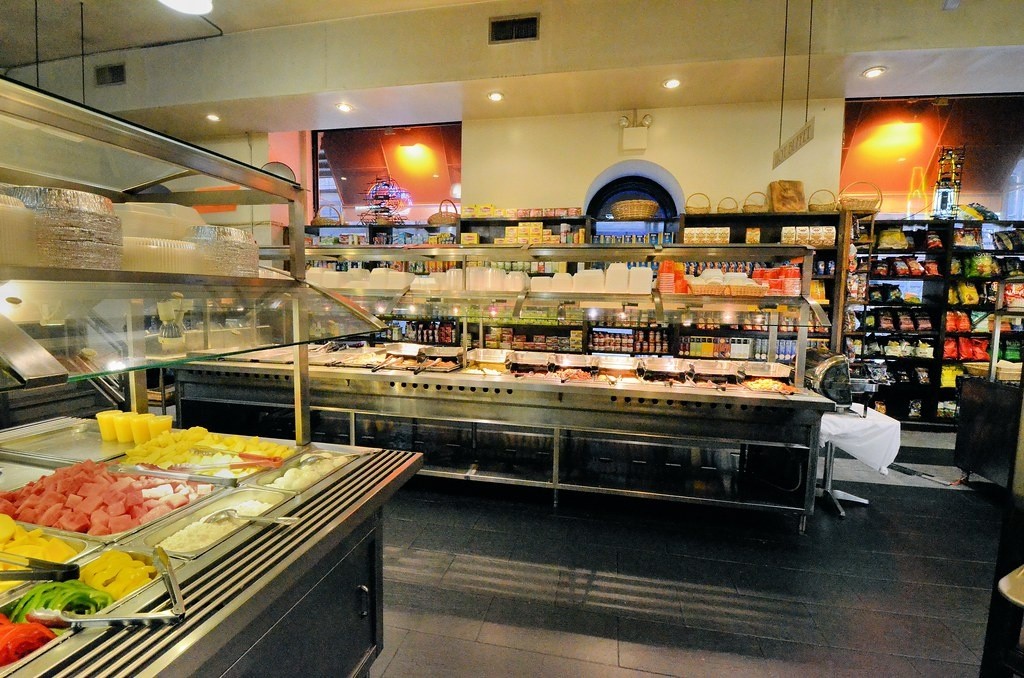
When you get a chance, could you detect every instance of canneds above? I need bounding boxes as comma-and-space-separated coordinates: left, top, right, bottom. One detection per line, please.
560, 228, 585, 244
588, 330, 668, 352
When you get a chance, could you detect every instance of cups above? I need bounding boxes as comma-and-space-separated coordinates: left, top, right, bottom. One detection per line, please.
95, 410, 173, 445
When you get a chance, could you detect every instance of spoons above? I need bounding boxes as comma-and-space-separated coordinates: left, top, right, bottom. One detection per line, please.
203, 508, 298, 526
297, 454, 328, 466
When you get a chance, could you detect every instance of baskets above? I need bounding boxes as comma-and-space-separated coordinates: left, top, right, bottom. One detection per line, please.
716, 197, 739, 214
742, 191, 770, 213
311, 204, 342, 227
808, 188, 837, 214
610, 199, 660, 221
838, 180, 882, 210
683, 192, 711, 214
427, 199, 461, 226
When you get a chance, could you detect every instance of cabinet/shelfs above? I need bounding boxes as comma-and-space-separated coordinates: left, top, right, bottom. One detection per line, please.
282, 143, 1024, 433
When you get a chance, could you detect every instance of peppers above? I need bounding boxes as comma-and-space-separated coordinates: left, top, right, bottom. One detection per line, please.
0, 551, 163, 669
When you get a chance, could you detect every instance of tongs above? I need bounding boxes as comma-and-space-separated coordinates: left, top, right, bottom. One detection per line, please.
560, 369, 579, 383
326, 355, 354, 367
0, 551, 80, 582
25, 546, 186, 629
414, 357, 442, 375
707, 379, 728, 392
371, 355, 404, 372
108, 464, 238, 488
170, 444, 284, 473
606, 374, 621, 387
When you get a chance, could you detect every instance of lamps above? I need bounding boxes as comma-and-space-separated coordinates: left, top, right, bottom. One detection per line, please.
619, 114, 653, 150
384, 129, 395, 135
932, 97, 948, 106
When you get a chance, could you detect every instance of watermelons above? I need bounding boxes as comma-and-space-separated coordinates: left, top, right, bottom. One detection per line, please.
0, 457, 211, 537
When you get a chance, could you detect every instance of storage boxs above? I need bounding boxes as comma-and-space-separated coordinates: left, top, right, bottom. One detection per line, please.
505, 208, 517, 217
517, 237, 529, 244
519, 222, 531, 227
517, 208, 530, 217
461, 233, 480, 244
661, 232, 674, 244
543, 229, 552, 236
542, 235, 550, 242
555, 208, 568, 216
505, 227, 517, 237
695, 227, 706, 245
796, 226, 809, 245
647, 233, 661, 245
706, 228, 718, 244
781, 227, 795, 244
568, 208, 581, 216
717, 227, 730, 244
531, 222, 543, 228
461, 204, 478, 217
530, 226, 542, 237
634, 235, 646, 244
493, 209, 506, 217
478, 204, 493, 217
822, 226, 836, 247
745, 228, 760, 243
517, 227, 530, 237
622, 235, 634, 243
505, 237, 518, 243
494, 238, 505, 245
550, 235, 560, 241
543, 208, 555, 216
684, 228, 695, 244
529, 208, 543, 217
810, 226, 822, 247
